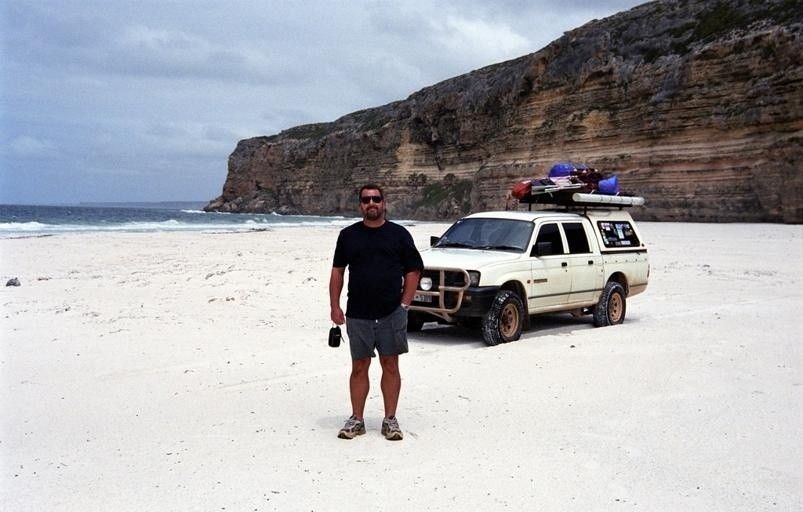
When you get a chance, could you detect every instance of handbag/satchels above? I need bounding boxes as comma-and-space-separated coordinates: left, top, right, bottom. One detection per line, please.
570, 169, 602, 182
512, 181, 532, 197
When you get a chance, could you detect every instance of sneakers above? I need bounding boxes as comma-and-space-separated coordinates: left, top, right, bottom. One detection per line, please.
337, 416, 365, 438
381, 416, 403, 440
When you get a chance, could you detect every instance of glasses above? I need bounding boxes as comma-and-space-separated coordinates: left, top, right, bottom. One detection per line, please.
360, 196, 381, 204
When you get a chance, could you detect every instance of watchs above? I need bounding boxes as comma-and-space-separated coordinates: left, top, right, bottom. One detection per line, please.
399, 303, 410, 312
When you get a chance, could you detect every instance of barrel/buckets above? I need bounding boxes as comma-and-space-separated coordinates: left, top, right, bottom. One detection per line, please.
598, 175, 618, 195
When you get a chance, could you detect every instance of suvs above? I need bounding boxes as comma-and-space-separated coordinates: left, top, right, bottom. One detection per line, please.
400, 210, 650, 345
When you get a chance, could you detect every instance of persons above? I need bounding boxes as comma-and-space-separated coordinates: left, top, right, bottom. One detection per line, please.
328, 184, 425, 440
